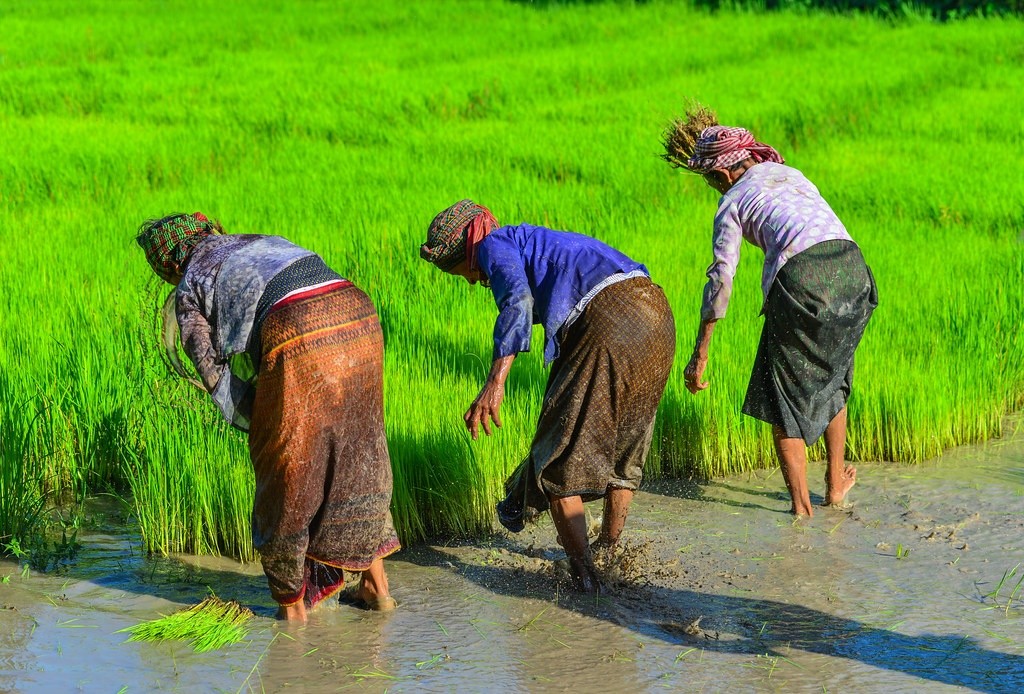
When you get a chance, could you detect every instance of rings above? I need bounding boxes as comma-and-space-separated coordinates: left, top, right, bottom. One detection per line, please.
684, 379, 689, 382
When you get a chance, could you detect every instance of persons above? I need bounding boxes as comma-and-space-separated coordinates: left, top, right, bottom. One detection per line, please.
656, 108, 879, 518
420, 199, 676, 592
137, 212, 402, 620
